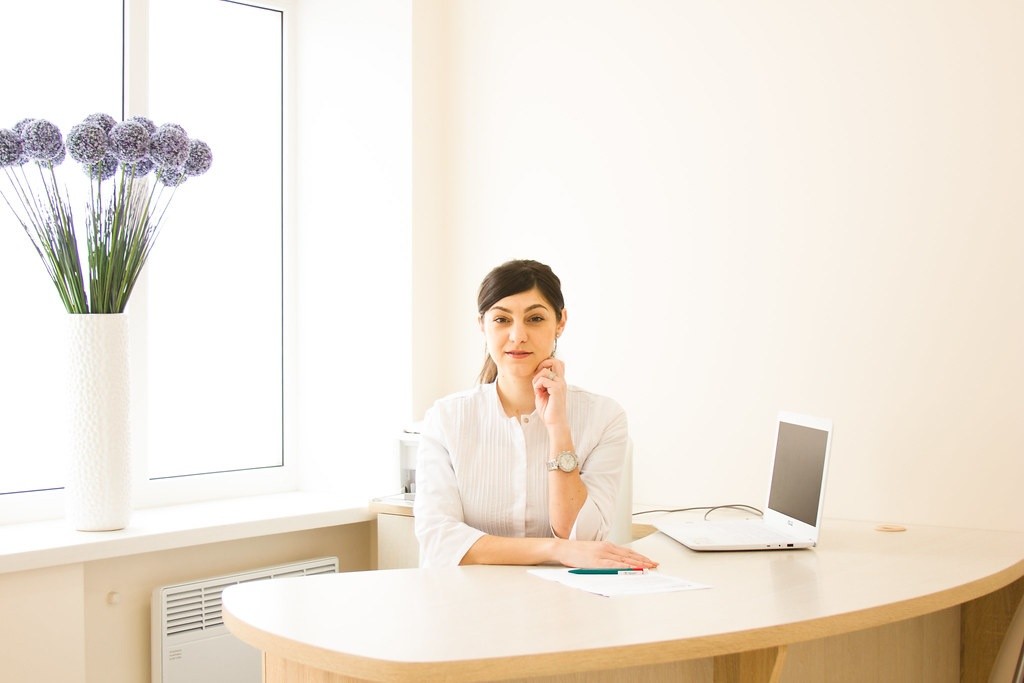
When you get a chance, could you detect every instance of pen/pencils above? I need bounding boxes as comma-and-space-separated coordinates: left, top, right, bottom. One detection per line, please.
568, 568, 649, 576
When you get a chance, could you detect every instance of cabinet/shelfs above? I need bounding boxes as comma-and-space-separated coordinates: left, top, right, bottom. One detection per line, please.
367, 492, 422, 571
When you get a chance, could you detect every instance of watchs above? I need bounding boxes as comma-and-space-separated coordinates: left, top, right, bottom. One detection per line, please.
547, 452, 580, 472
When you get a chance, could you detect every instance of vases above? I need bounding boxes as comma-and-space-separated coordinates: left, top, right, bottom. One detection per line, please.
68, 313, 132, 533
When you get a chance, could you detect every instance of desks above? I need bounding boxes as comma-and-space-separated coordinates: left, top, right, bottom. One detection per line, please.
210, 525, 1024, 683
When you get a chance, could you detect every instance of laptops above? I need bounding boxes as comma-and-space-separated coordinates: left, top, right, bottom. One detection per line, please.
652, 410, 833, 551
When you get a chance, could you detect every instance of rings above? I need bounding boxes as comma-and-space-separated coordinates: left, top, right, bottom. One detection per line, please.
548, 372, 557, 380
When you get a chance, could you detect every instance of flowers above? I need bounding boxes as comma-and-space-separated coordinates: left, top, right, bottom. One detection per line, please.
0, 114, 216, 310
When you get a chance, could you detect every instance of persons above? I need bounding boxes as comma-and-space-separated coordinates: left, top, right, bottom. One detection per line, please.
413, 259, 660, 569
